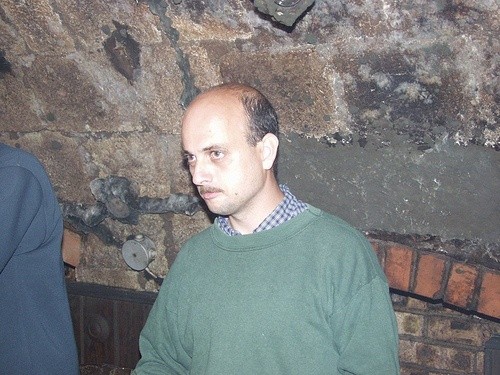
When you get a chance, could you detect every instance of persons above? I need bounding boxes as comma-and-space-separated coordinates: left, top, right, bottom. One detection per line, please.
0, 143, 81, 375
124, 82, 400, 375
